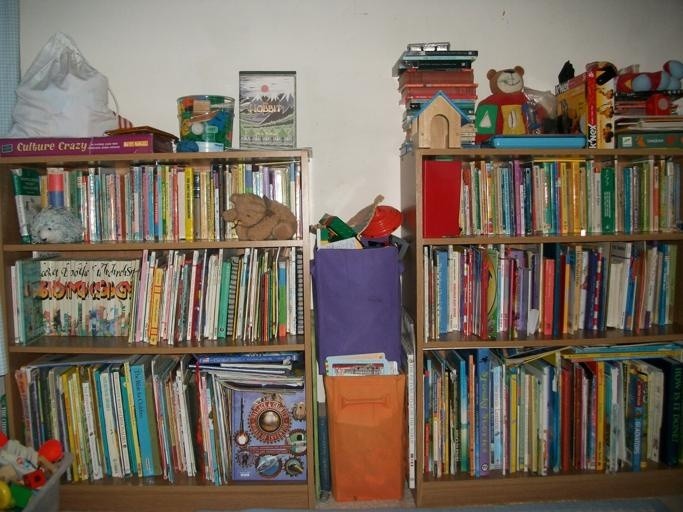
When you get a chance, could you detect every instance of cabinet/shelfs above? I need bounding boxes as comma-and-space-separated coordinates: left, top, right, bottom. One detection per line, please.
401, 149, 683, 508
0, 149, 318, 510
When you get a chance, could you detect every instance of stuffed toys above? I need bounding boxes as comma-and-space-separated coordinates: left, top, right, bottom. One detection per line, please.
477, 66, 538, 144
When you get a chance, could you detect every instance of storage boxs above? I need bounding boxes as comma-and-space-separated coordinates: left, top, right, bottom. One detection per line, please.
0, 438, 72, 511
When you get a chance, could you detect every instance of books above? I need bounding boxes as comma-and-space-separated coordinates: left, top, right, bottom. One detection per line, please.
423, 244, 678, 341
237, 68, 294, 150
11, 249, 305, 344
393, 54, 477, 146
7, 351, 308, 485
424, 161, 683, 239
323, 351, 399, 378
555, 71, 683, 149
11, 163, 302, 243
423, 340, 683, 477
